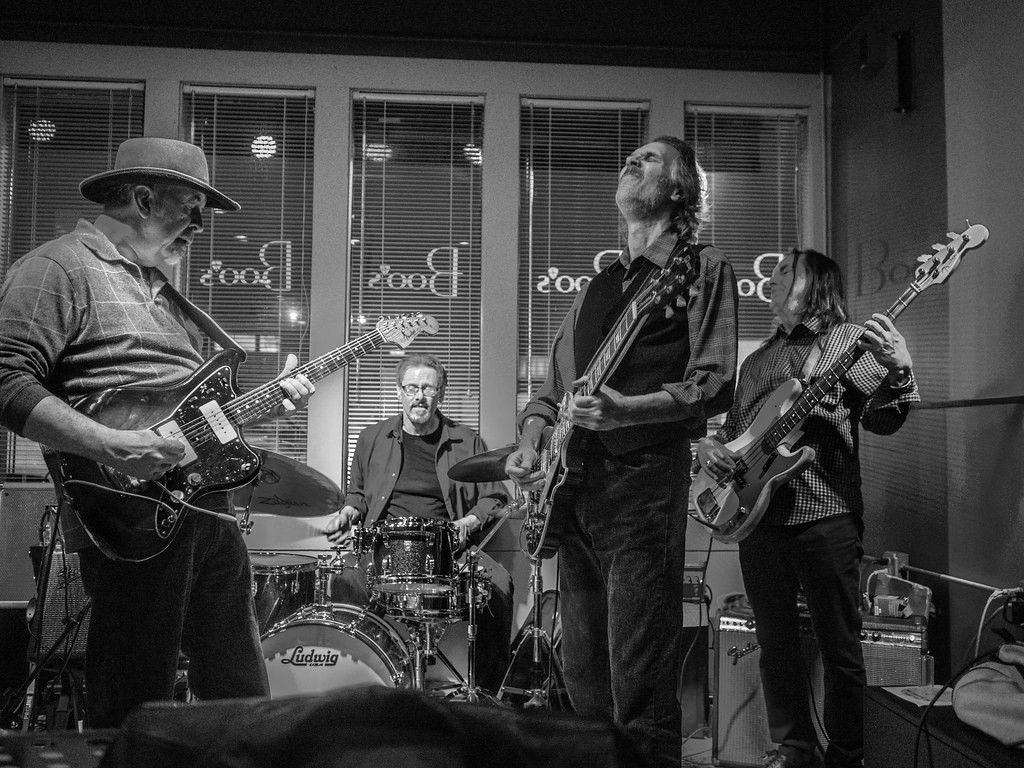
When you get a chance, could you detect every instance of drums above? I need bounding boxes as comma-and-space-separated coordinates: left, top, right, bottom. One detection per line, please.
370, 516, 455, 598
384, 563, 487, 622
249, 551, 319, 637
257, 602, 416, 699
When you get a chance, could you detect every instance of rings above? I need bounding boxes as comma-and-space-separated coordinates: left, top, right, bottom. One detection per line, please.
705, 460, 711, 466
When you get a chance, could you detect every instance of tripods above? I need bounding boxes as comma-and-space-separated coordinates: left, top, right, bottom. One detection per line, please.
440, 488, 574, 722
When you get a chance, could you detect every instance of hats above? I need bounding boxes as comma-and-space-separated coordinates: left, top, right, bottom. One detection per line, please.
78, 138, 242, 211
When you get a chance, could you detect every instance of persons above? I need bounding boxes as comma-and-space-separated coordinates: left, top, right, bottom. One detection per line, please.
692, 248, 920, 768
505, 137, 737, 768
326, 356, 527, 706
0, 139, 316, 730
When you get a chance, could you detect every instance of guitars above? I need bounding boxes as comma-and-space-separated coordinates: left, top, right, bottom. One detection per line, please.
52, 311, 440, 566
524, 243, 703, 559
689, 215, 992, 545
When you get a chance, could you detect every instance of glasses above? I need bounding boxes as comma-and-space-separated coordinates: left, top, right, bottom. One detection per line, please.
398, 380, 444, 397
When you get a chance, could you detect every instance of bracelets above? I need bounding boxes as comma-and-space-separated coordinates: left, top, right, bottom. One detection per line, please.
889, 375, 912, 389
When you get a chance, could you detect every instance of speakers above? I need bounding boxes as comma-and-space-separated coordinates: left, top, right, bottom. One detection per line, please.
711, 607, 926, 768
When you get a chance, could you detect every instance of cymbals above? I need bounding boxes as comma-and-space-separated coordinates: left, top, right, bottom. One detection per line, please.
447, 443, 520, 483
228, 446, 346, 518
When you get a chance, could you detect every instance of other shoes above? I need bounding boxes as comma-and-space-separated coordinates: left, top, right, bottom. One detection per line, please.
763, 750, 798, 768
